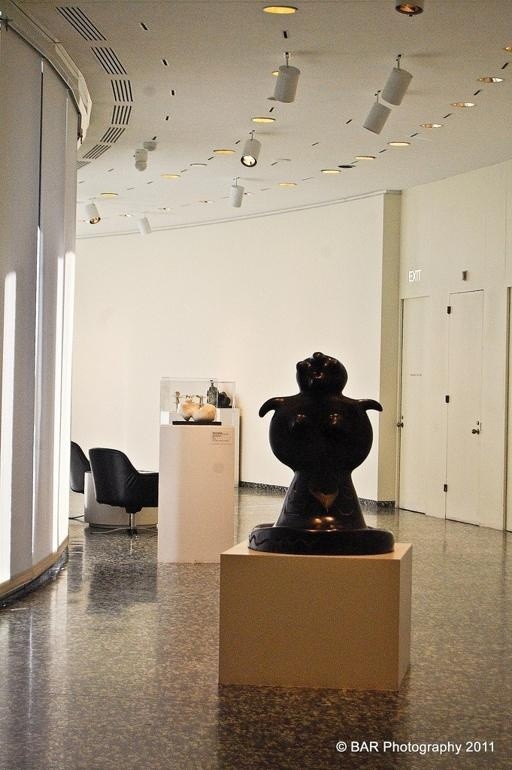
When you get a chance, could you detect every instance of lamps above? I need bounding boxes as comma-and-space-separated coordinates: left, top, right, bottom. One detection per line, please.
273, 52, 301, 104
230, 130, 263, 208
361, 53, 414, 136
84, 197, 101, 225
395, 0, 425, 16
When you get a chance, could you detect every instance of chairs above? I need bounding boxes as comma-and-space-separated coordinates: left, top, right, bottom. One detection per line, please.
69, 441, 158, 538
